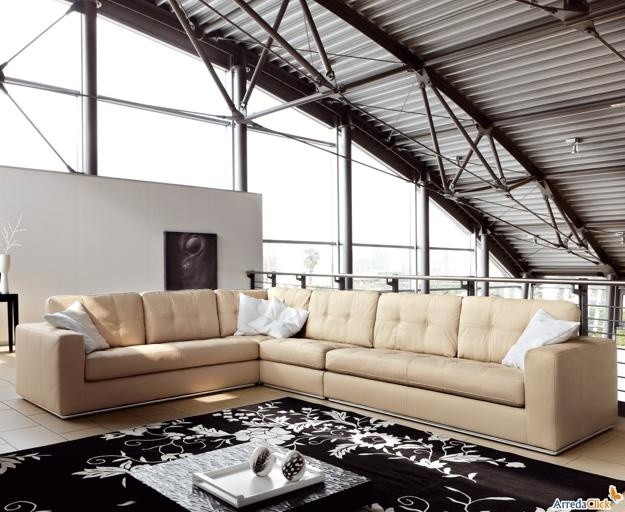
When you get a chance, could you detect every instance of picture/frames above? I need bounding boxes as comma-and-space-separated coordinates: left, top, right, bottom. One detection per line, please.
164, 230, 217, 290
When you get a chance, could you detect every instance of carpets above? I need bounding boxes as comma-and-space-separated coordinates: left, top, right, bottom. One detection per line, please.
0, 396, 625, 512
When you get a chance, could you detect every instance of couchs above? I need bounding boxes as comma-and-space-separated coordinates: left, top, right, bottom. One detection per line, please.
15, 286, 618, 458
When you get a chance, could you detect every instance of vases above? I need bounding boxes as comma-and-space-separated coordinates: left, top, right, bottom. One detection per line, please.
0, 254, 11, 295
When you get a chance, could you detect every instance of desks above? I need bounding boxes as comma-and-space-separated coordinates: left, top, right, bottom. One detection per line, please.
0, 294, 19, 354
